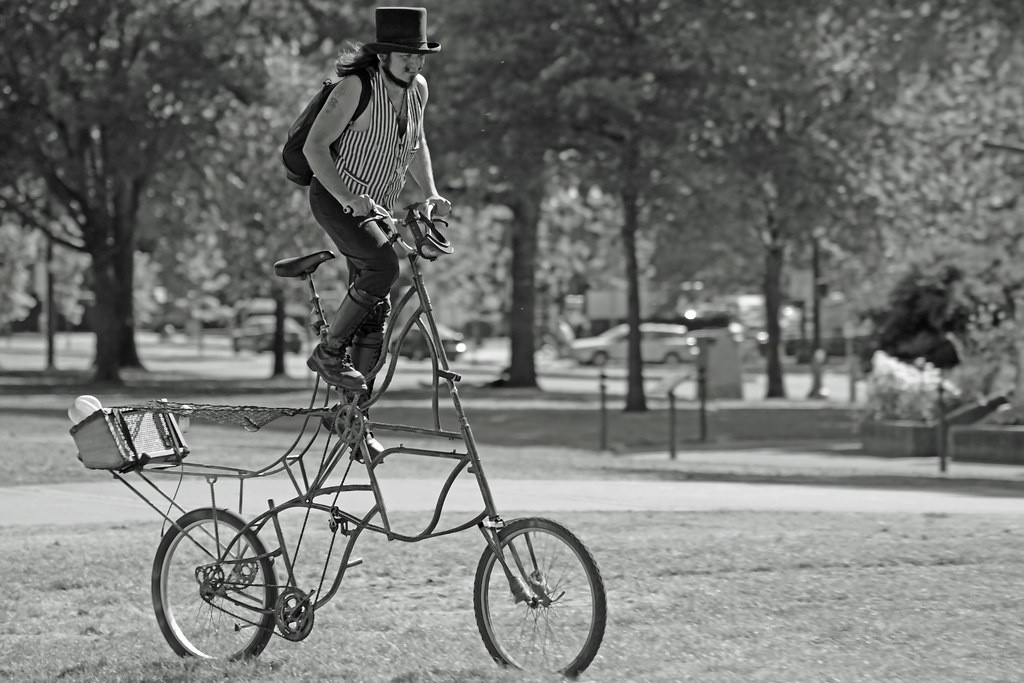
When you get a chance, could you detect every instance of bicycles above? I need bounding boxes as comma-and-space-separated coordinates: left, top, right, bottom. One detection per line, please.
65, 197, 608, 681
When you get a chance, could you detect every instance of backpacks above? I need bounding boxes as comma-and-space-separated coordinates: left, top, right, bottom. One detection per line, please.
281, 66, 372, 186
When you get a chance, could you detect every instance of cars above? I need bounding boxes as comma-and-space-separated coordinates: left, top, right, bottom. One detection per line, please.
571, 320, 701, 366
141, 287, 309, 355
384, 317, 466, 360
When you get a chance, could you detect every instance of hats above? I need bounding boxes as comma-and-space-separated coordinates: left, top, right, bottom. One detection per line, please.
363, 6, 441, 54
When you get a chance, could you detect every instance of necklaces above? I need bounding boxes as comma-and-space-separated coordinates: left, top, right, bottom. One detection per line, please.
382, 80, 408, 136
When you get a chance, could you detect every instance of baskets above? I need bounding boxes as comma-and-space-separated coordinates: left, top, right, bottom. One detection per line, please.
70, 397, 191, 473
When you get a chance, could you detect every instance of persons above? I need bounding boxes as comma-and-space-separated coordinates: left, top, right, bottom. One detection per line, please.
294, 4, 452, 461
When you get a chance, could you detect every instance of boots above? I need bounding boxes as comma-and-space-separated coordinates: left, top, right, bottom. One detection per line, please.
307, 276, 384, 464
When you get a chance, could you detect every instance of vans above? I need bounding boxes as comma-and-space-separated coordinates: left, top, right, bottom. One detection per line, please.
670, 311, 745, 358
